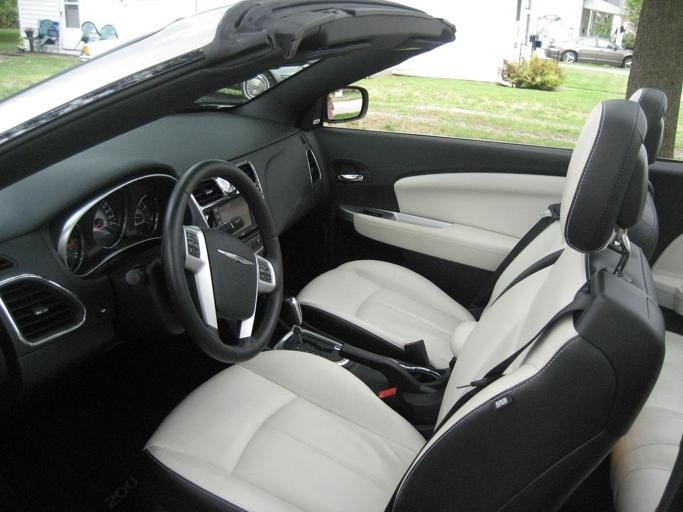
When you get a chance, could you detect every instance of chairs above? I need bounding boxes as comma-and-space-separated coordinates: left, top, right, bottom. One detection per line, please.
145, 98, 667, 512
289, 87, 670, 424
603, 329, 683, 512
649, 233, 682, 322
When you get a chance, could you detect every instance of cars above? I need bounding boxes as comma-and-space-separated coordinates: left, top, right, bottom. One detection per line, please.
76, 14, 346, 103
544, 35, 634, 69
